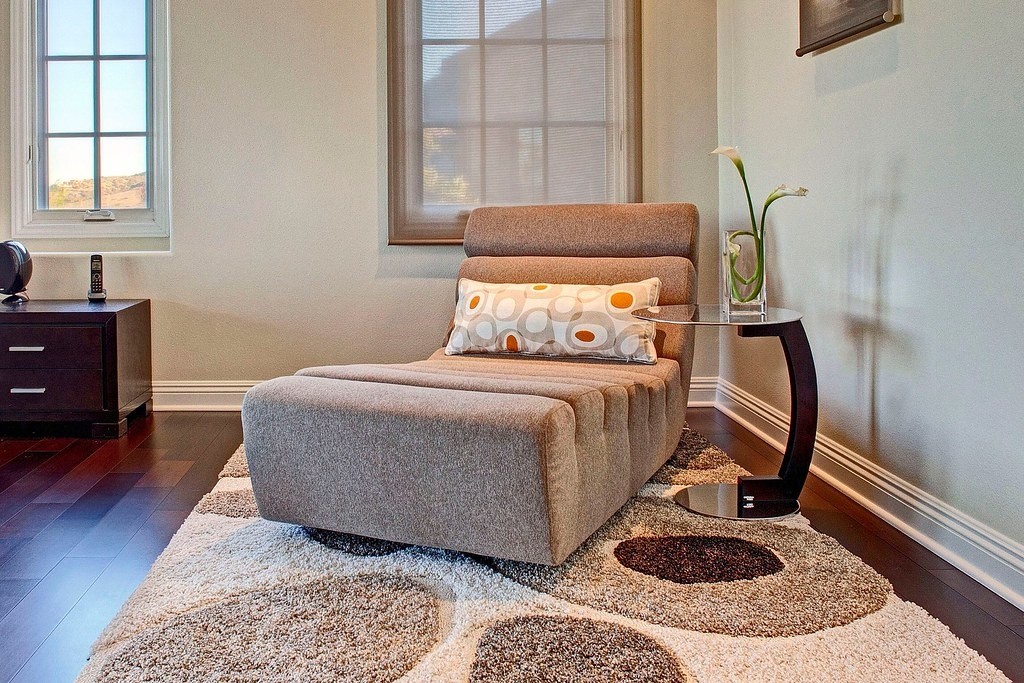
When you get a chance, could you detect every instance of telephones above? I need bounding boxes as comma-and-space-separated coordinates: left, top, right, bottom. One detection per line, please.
90, 254, 104, 294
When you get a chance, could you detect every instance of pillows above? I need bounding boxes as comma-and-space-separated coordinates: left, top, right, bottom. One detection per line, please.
443, 277, 664, 366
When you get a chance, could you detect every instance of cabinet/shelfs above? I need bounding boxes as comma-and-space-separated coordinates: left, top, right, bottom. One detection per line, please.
0, 298, 155, 438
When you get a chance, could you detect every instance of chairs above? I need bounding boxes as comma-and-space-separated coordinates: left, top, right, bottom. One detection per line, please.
239, 201, 701, 566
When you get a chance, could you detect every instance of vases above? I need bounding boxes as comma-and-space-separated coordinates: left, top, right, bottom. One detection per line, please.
720, 229, 769, 323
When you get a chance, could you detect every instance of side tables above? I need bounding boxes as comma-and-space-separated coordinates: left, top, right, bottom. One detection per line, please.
629, 301, 818, 521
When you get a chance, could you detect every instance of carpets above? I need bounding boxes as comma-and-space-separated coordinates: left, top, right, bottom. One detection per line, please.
73, 420, 1015, 682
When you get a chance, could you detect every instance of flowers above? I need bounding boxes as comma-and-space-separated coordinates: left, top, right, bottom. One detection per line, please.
710, 146, 810, 303
